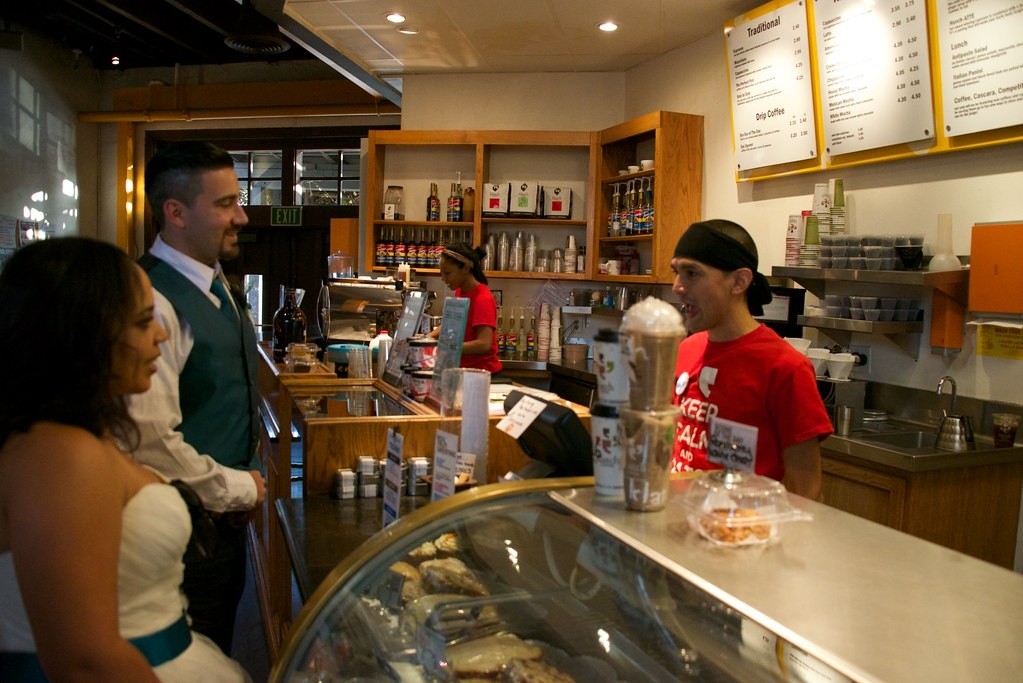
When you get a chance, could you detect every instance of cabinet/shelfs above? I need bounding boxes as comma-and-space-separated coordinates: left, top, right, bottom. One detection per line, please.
253, 111, 704, 669
772, 264, 969, 360
814, 445, 1022, 568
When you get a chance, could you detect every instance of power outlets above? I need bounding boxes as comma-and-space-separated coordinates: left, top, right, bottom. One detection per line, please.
848, 345, 871, 373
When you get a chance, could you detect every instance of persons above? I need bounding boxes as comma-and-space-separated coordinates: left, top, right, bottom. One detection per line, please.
424, 242, 502, 378
117, 140, 267, 659
0, 238, 248, 683
670, 219, 834, 501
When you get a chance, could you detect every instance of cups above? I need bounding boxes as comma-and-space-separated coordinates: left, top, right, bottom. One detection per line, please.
620, 295, 686, 412
824, 294, 924, 322
345, 391, 370, 417
461, 371, 490, 487
537, 303, 562, 361
401, 336, 439, 402
483, 229, 586, 274
594, 328, 631, 403
785, 177, 846, 266
618, 159, 653, 176
821, 234, 934, 269
834, 405, 853, 437
599, 241, 646, 310
956, 255, 972, 267
348, 339, 393, 379
991, 412, 1021, 447
616, 403, 683, 513
590, 401, 628, 504
783, 337, 859, 382
441, 368, 488, 417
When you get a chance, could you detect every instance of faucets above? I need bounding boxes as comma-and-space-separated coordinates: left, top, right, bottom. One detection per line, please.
937, 375, 957, 413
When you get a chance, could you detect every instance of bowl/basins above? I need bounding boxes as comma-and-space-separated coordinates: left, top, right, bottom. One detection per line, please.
671, 470, 801, 547
284, 342, 321, 373
420, 474, 478, 498
563, 344, 589, 360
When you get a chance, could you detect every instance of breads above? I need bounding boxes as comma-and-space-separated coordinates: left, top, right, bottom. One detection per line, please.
388, 534, 577, 683
700, 508, 771, 542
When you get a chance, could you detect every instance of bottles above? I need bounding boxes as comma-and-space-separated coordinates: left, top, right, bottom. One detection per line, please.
370, 331, 393, 377
607, 176, 653, 236
273, 285, 307, 362
373, 171, 476, 287
565, 286, 619, 307
496, 305, 536, 358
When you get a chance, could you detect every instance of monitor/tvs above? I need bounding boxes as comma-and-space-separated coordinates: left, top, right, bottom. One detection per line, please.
502, 389, 595, 478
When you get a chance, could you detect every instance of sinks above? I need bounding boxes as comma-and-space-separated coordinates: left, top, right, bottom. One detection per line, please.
864, 431, 940, 450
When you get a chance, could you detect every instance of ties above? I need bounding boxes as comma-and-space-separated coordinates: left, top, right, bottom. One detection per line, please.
209, 276, 239, 323
